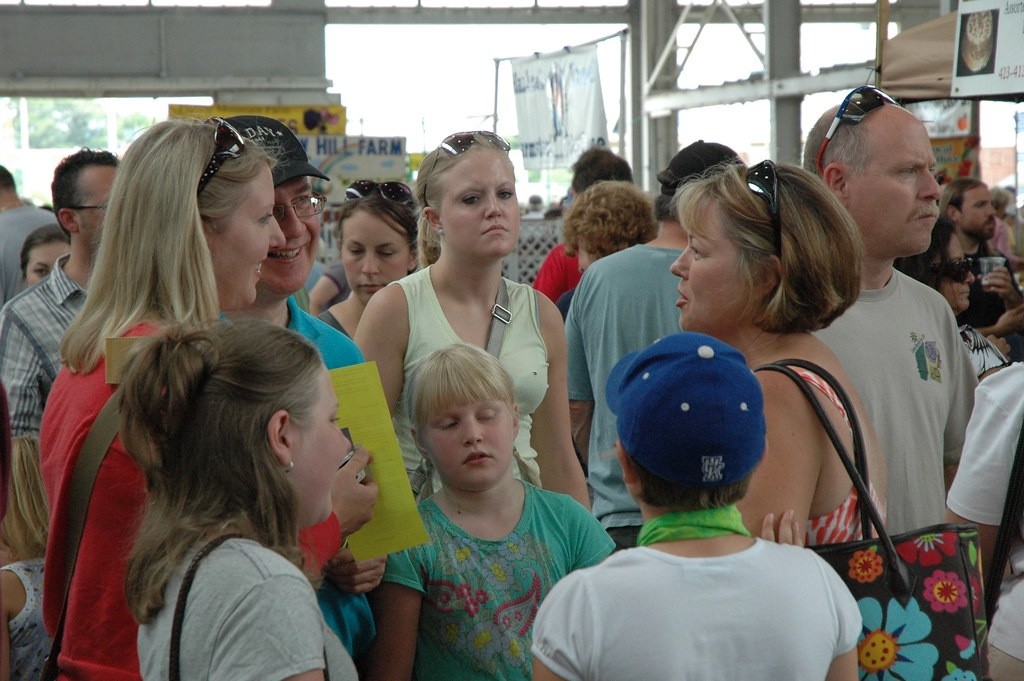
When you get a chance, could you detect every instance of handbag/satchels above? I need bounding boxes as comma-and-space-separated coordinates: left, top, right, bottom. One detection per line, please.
755, 358, 992, 681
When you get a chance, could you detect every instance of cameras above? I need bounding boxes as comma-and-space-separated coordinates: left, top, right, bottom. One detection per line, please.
337, 426, 366, 482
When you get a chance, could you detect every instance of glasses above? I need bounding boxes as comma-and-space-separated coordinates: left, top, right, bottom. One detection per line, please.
423, 132, 511, 217
346, 179, 416, 208
193, 117, 246, 203
748, 159, 782, 258
272, 189, 328, 224
815, 85, 898, 176
939, 256, 974, 285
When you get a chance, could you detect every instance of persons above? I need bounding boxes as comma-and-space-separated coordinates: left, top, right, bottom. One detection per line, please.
799, 88, 980, 532
530, 334, 861, 681
350, 130, 592, 681
0, 149, 146, 535
184, 117, 432, 681
122, 318, 359, 680
381, 345, 618, 681
0, 86, 1023, 681
41, 116, 284, 681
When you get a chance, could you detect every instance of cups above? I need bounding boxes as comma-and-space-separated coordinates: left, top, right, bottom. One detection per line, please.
979, 257, 1005, 291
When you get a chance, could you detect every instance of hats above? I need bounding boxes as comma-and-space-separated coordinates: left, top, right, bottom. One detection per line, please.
231, 116, 329, 190
660, 142, 745, 195
604, 331, 768, 483
